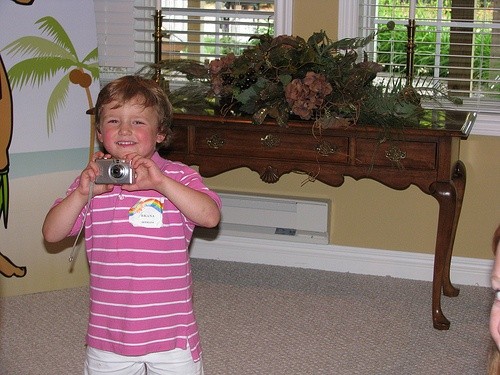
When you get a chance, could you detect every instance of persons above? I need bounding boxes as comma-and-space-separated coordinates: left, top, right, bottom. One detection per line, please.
42, 74, 224, 375
486, 224, 500, 375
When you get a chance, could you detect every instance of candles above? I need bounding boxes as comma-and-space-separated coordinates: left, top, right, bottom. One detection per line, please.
156, 0, 161, 10
409, 0, 416, 19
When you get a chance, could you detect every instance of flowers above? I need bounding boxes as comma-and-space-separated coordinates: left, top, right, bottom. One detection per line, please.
207, 52, 238, 99
283, 71, 332, 121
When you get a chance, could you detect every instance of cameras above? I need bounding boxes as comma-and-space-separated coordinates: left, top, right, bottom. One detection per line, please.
94, 158, 136, 185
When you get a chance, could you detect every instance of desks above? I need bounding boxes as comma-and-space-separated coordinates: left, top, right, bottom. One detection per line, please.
82, 101, 478, 332
